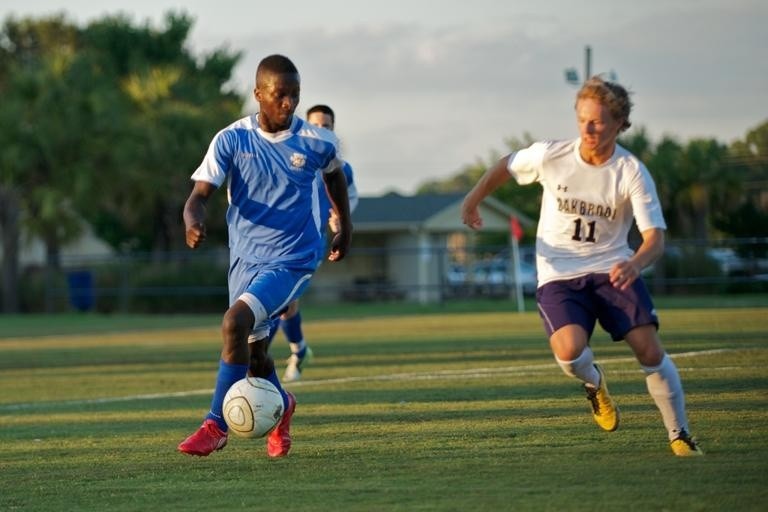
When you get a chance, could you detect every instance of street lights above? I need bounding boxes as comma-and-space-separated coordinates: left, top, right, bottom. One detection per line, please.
563, 45, 621, 92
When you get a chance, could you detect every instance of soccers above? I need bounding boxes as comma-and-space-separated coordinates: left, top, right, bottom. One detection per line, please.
223, 377, 286, 438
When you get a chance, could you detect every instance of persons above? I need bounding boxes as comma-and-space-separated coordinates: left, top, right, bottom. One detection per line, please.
177, 53, 353, 457
277, 105, 360, 383
461, 76, 705, 458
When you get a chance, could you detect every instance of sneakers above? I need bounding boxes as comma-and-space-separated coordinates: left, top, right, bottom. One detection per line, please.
584, 363, 620, 432
177, 418, 228, 456
282, 346, 313, 384
267, 391, 296, 457
670, 427, 704, 457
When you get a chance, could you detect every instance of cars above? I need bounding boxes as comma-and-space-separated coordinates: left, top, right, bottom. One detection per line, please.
448, 235, 746, 294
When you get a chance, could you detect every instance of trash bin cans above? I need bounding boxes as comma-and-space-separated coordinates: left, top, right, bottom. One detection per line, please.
66, 270, 96, 313
